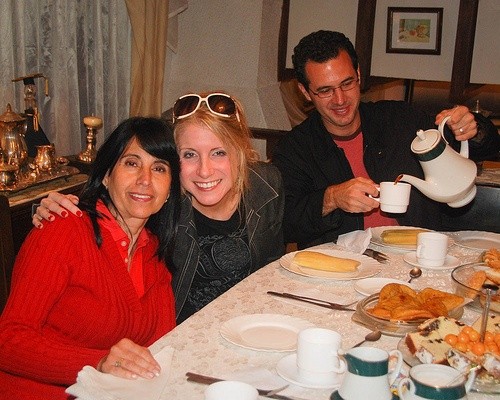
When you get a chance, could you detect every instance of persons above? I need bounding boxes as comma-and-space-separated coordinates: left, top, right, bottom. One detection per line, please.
272, 30, 500, 254
0, 95, 280, 400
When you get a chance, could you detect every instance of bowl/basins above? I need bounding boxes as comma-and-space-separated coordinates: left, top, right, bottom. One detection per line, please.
204, 380, 259, 400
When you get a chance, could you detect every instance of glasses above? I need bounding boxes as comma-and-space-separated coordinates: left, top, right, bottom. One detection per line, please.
173, 93, 242, 128
309, 79, 359, 98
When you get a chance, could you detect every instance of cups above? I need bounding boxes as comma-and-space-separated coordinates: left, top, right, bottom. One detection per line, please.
0, 168, 18, 187
369, 181, 412, 214
416, 232, 448, 267
398, 363, 468, 400
34, 142, 55, 172
297, 327, 346, 385
338, 348, 403, 400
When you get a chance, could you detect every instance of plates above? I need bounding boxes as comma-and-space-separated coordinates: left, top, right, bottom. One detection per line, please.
396, 332, 500, 394
367, 225, 436, 251
403, 252, 460, 270
275, 353, 346, 388
280, 249, 383, 281
329, 384, 400, 400
452, 230, 500, 252
450, 261, 500, 317
356, 293, 464, 334
219, 314, 315, 352
353, 278, 415, 297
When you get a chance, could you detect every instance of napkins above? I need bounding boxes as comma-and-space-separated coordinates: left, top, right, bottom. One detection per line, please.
65, 343, 174, 400
330, 228, 373, 255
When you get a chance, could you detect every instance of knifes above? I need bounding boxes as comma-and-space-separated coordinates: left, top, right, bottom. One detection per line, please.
267, 291, 356, 311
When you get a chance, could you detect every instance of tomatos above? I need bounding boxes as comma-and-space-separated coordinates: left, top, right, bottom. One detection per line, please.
444, 325, 500, 359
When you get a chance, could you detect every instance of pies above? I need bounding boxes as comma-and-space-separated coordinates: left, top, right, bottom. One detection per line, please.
365, 283, 466, 321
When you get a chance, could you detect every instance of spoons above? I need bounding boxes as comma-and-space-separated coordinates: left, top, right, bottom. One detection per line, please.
479, 284, 499, 344
407, 267, 422, 283
351, 330, 382, 348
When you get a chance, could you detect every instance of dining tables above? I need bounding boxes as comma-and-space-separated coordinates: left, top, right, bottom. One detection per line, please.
75, 229, 500, 400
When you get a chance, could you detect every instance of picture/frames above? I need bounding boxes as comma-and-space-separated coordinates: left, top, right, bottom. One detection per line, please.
386, 7, 444, 55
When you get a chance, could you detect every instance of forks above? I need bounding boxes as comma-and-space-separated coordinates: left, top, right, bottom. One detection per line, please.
283, 293, 358, 308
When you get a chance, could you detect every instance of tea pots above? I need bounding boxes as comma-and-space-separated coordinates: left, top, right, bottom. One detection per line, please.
398, 117, 478, 209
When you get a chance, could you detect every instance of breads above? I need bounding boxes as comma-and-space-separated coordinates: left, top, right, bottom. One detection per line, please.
293, 251, 362, 272
405, 311, 500, 380
380, 229, 430, 245
464, 249, 500, 312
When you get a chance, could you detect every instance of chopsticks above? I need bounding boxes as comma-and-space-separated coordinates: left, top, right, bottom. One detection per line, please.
185, 372, 292, 400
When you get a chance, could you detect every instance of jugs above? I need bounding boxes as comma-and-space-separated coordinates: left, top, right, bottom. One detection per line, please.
0, 103, 28, 174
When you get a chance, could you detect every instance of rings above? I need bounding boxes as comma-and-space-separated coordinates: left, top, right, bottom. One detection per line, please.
459, 127, 464, 136
114, 360, 122, 367
